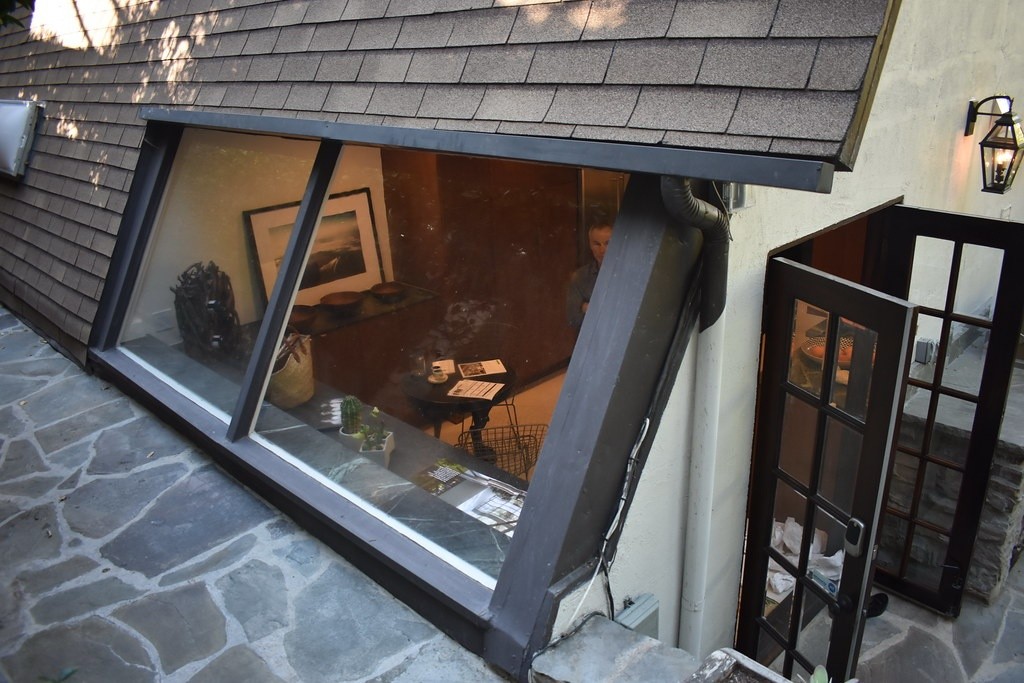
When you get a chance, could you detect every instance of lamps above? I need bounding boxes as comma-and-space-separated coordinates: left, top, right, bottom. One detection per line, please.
965, 96, 1024, 195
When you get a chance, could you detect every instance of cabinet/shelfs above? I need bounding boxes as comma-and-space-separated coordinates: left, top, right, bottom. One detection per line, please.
172, 281, 527, 538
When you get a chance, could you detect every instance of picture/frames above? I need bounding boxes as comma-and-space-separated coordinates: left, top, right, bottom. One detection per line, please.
243, 187, 385, 316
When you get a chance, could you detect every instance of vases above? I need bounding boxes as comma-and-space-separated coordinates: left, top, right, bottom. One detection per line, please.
359, 431, 394, 469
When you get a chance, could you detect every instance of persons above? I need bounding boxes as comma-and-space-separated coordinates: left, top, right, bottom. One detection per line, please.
564, 219, 614, 330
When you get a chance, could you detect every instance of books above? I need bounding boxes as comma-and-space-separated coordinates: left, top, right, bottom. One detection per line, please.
458, 359, 507, 378
447, 378, 505, 400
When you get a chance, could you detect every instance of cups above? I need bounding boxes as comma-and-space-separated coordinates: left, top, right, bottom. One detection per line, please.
433, 369, 443, 379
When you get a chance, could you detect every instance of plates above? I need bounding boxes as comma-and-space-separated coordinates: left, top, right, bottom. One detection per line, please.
428, 375, 448, 384
802, 338, 854, 367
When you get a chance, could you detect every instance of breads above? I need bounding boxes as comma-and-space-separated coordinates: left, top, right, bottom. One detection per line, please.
809, 345, 852, 362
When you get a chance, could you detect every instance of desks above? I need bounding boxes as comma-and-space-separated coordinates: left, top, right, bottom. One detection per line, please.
401, 352, 519, 439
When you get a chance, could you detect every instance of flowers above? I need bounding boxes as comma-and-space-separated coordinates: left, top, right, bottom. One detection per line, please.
353, 406, 385, 451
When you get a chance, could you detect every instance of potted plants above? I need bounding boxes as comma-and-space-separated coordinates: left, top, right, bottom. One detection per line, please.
339, 394, 368, 452
